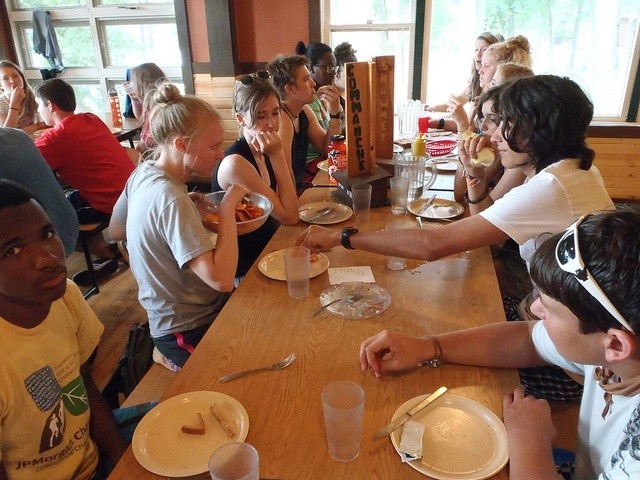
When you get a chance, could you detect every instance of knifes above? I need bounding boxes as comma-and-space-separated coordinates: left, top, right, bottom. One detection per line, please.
417, 193, 438, 214
376, 386, 448, 439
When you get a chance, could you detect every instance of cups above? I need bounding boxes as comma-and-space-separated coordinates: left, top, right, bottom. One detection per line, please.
398, 100, 422, 137
284, 248, 310, 298
390, 176, 407, 215
322, 380, 365, 461
208, 442, 259, 480
419, 116, 430, 135
352, 183, 371, 220
384, 223, 410, 271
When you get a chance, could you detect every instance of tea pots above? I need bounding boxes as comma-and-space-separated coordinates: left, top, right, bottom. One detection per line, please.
394, 155, 437, 202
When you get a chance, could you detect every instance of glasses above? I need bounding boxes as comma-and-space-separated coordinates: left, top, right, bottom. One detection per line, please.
313, 65, 339, 74
555, 211, 635, 337
234, 70, 272, 112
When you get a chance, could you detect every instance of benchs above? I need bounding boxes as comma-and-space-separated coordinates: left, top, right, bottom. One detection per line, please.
117, 360, 180, 410
77, 213, 102, 301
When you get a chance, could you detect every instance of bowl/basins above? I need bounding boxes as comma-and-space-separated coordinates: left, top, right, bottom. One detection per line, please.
194, 190, 274, 236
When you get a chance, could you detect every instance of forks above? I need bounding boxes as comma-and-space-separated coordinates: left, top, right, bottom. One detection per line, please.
219, 352, 297, 383
313, 292, 364, 317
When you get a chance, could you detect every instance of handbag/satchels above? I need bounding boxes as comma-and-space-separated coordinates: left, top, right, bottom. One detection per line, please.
116, 321, 155, 398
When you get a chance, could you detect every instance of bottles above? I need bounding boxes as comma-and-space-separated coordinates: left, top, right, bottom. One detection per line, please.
328, 134, 346, 179
107, 87, 123, 128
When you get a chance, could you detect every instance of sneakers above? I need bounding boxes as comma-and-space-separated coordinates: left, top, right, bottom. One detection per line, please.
109, 241, 129, 265
72, 258, 117, 283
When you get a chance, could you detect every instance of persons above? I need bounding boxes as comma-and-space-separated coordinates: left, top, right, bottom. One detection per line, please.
266, 53, 344, 196
295, 39, 339, 192
0, 60, 36, 128
480, 77, 528, 203
293, 75, 616, 403
334, 41, 358, 133
358, 204, 640, 480
109, 83, 250, 368
122, 62, 169, 168
0, 128, 79, 259
33, 78, 136, 266
210, 70, 299, 288
1, 178, 131, 478
425, 31, 505, 113
447, 62, 535, 204
418, 34, 533, 133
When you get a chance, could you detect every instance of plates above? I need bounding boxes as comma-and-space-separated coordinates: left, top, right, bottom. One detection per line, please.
132, 390, 249, 478
258, 247, 329, 281
426, 157, 461, 171
298, 200, 354, 225
34, 128, 50, 138
390, 393, 510, 479
319, 282, 392, 319
422, 132, 459, 145
407, 197, 465, 219
109, 126, 121, 135
317, 160, 329, 172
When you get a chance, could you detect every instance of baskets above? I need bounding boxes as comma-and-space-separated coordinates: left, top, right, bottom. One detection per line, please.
425, 139, 458, 156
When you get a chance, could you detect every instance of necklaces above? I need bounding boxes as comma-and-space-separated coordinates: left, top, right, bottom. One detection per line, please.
281, 101, 300, 120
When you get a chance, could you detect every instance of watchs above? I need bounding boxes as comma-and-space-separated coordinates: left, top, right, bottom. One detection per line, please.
341, 224, 359, 251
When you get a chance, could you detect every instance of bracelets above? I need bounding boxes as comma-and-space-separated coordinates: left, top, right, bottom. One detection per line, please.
423, 333, 443, 368
8, 106, 20, 112
463, 190, 488, 205
455, 130, 471, 133
328, 111, 342, 120
437, 117, 444, 129
458, 168, 485, 194
